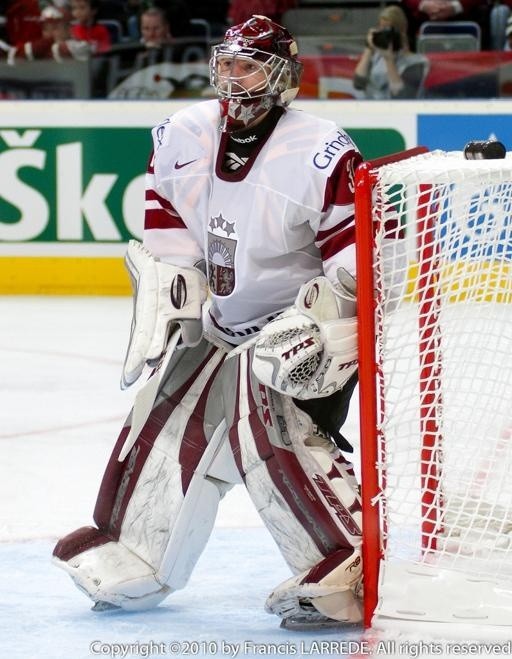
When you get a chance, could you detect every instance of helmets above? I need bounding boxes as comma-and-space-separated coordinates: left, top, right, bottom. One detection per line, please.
208, 15, 303, 106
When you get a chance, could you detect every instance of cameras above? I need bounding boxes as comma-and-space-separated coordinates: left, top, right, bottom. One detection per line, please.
371, 26, 402, 51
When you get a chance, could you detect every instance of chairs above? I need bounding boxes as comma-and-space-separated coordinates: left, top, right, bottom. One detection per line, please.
191, 20, 211, 54
95, 17, 121, 44
415, 20, 483, 57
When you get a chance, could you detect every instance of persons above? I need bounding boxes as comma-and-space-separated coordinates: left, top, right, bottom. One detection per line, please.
0, 0, 512, 99
50, 13, 385, 629
